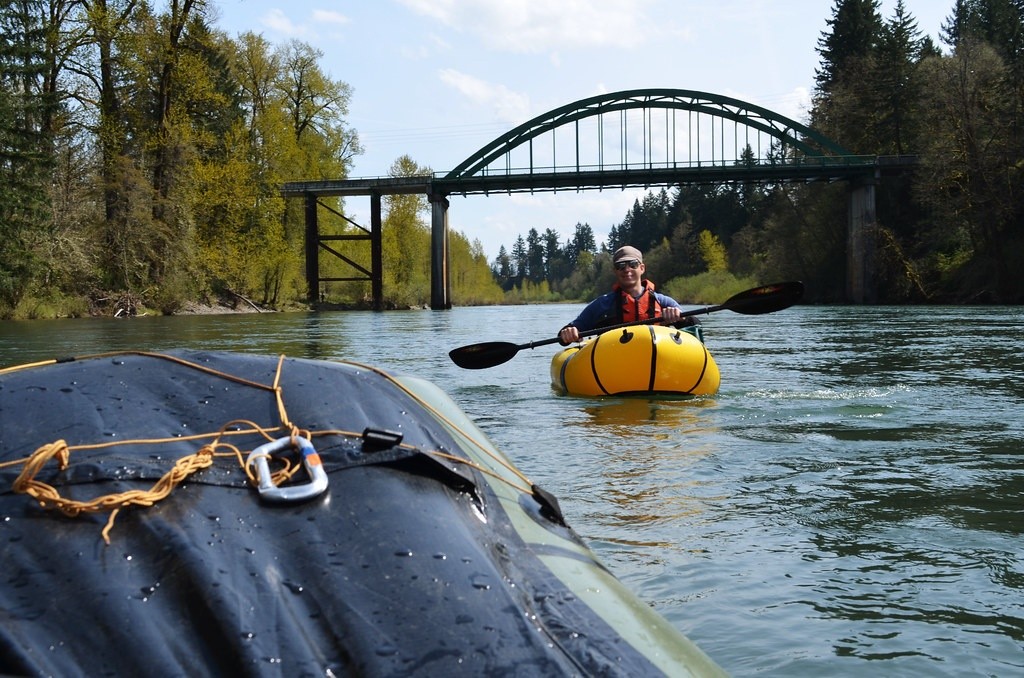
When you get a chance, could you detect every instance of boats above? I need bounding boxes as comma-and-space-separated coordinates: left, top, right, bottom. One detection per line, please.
551, 316, 723, 401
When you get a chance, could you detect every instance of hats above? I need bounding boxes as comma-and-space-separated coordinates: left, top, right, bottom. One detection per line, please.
612, 245, 643, 265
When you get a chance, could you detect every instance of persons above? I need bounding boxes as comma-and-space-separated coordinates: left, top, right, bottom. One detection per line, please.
558, 246, 701, 347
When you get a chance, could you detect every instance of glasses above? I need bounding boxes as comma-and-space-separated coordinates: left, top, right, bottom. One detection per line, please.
614, 260, 640, 271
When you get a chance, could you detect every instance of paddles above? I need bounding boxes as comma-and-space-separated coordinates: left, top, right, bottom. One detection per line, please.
447, 280, 807, 371
